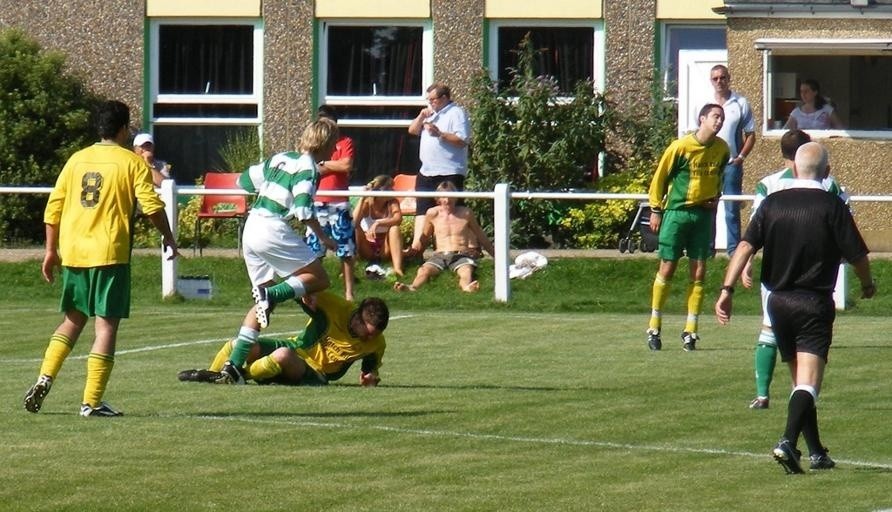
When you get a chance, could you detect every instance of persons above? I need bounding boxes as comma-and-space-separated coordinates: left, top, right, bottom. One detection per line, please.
349, 174, 405, 277
739, 128, 850, 409
646, 104, 730, 351
22, 100, 178, 418
178, 290, 389, 388
132, 132, 172, 188
404, 80, 470, 257
393, 180, 494, 293
782, 77, 845, 130
694, 64, 756, 259
220, 118, 341, 386
305, 104, 357, 302
714, 141, 877, 475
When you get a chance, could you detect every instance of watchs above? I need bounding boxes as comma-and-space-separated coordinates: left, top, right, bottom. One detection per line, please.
319, 160, 324, 166
738, 154, 746, 161
720, 285, 735, 294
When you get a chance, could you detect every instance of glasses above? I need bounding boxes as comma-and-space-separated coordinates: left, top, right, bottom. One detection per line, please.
713, 76, 726, 82
425, 97, 440, 101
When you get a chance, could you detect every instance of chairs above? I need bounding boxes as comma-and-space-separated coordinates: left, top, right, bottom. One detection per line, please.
390, 174, 418, 217
192, 172, 247, 258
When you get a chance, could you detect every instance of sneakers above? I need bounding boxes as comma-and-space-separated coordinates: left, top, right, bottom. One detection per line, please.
179, 370, 196, 380
646, 327, 661, 350
192, 369, 217, 379
810, 448, 835, 472
773, 437, 806, 476
80, 402, 123, 417
682, 331, 700, 352
223, 360, 245, 383
253, 285, 275, 328
24, 374, 52, 412
750, 395, 769, 410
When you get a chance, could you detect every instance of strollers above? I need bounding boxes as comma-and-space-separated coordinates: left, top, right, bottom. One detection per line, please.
619, 200, 666, 254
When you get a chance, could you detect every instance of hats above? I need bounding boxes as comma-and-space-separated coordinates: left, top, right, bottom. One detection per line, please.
134, 133, 154, 148
